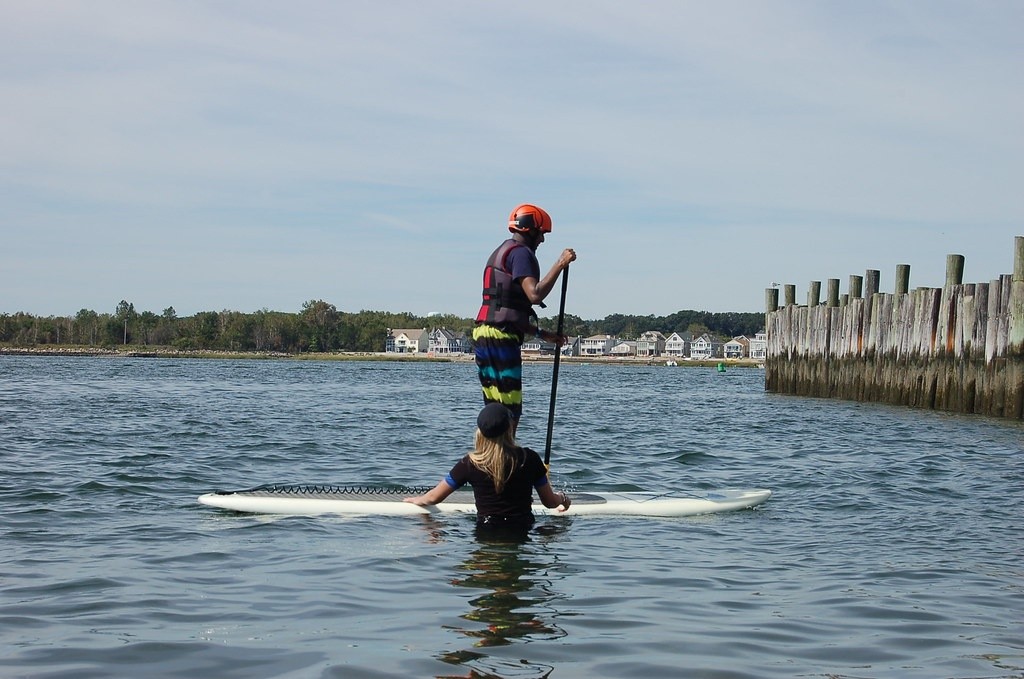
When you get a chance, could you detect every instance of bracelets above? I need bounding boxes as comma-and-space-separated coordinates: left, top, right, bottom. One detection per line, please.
559, 491, 568, 504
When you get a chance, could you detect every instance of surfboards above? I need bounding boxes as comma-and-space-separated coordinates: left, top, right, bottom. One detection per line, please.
198, 489, 769, 516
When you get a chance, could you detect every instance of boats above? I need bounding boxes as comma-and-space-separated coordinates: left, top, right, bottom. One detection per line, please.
666, 356, 677, 367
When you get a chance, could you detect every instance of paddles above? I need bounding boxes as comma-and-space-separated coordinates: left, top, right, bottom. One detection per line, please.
543, 265, 569, 472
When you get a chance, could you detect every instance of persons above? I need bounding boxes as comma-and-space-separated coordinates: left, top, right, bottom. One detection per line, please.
402, 403, 572, 540
472, 203, 576, 437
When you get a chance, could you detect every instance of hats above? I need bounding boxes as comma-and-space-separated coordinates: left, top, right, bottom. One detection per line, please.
477, 403, 513, 439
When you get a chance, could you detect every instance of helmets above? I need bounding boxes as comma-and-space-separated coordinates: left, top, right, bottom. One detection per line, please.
509, 204, 551, 233
